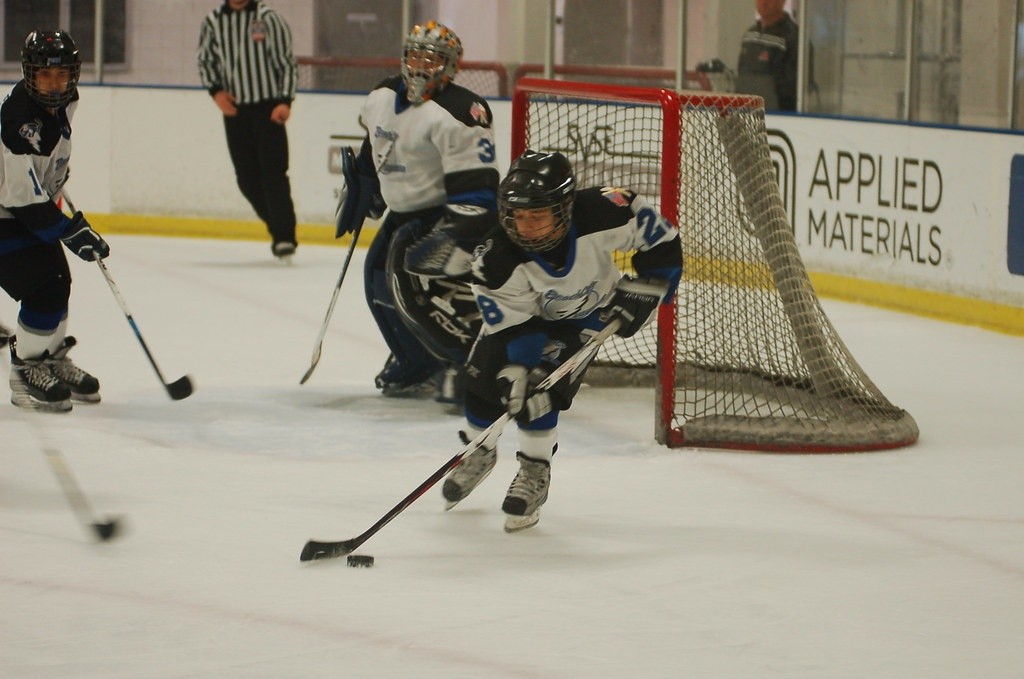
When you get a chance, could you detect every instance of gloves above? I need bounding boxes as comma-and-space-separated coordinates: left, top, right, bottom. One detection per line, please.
402, 203, 494, 278
496, 363, 552, 423
336, 178, 369, 240
61, 211, 111, 262
604, 273, 668, 339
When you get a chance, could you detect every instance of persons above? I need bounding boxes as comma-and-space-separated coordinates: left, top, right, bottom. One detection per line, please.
0, 30, 109, 414
194, 0, 301, 266
738, 0, 815, 112
334, 20, 499, 395
443, 149, 683, 532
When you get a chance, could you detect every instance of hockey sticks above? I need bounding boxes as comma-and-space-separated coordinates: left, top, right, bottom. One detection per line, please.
57, 191, 198, 401
300, 321, 625, 563
0, 336, 121, 543
299, 199, 372, 378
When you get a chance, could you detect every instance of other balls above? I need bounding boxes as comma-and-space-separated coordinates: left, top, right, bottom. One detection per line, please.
347, 555, 374, 567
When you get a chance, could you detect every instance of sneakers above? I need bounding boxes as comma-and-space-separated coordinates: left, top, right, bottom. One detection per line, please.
375, 353, 439, 398
502, 442, 558, 529
42, 336, 101, 403
270, 222, 296, 267
442, 431, 497, 511
9, 335, 73, 414
435, 360, 480, 415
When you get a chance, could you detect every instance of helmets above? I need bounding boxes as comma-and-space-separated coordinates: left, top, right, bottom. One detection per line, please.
500, 149, 574, 225
21, 28, 81, 98
404, 20, 464, 103
